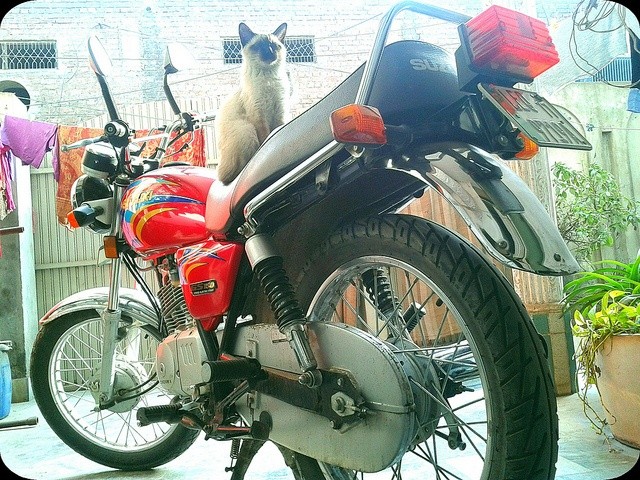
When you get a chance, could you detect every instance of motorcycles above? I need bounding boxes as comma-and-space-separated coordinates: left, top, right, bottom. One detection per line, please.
32, 0, 592, 480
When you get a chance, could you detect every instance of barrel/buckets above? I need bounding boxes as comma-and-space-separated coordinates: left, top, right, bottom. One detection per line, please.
0, 344, 13, 420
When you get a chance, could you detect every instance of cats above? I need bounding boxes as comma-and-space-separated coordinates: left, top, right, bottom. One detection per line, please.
213, 21, 292, 186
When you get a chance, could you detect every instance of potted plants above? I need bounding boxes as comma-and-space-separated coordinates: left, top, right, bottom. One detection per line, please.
559, 252, 640, 450
